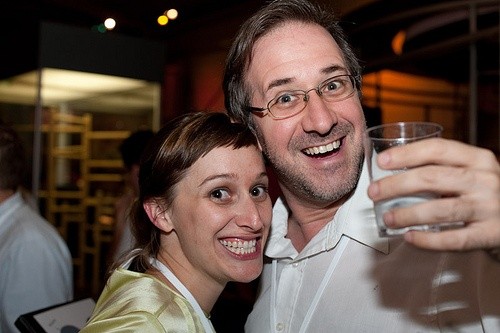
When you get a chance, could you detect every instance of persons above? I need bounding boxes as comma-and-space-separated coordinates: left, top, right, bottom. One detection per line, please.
0, 123, 164, 333
223, 0, 500, 333
78, 110, 273, 333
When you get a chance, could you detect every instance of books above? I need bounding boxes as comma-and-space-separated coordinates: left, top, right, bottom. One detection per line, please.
15, 297, 97, 333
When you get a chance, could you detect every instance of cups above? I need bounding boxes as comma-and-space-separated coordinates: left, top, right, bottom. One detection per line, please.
363, 122, 443, 238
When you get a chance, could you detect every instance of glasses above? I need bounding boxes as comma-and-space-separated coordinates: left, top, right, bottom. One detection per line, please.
244, 75, 361, 121
